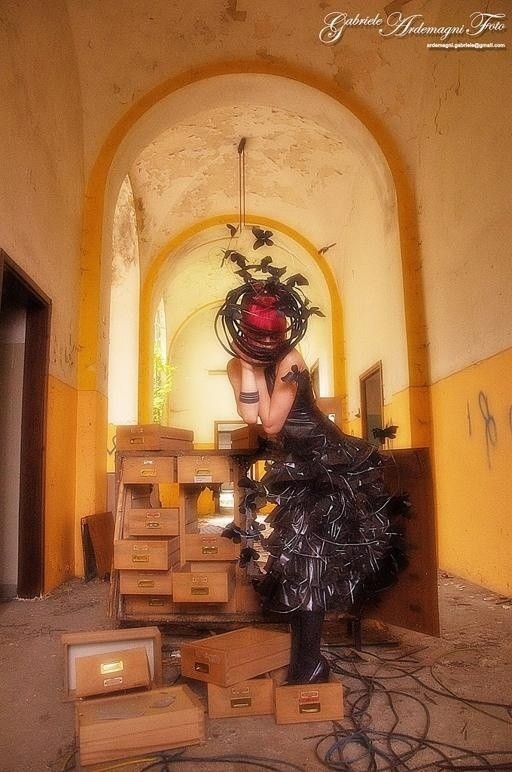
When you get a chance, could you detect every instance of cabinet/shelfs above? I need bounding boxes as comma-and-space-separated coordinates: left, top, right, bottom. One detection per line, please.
106, 424, 344, 626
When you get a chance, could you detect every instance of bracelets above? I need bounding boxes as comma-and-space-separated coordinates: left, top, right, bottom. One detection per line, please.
238, 390, 260, 405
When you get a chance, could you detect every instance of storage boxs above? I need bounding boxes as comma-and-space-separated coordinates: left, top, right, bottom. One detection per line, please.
60, 625, 164, 702
202, 672, 277, 719
179, 625, 293, 689
70, 683, 209, 766
264, 670, 344, 726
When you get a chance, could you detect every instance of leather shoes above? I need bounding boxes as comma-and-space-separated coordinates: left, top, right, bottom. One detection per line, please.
281, 654, 330, 686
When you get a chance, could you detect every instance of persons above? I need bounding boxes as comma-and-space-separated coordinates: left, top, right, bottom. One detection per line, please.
226, 286, 417, 686
